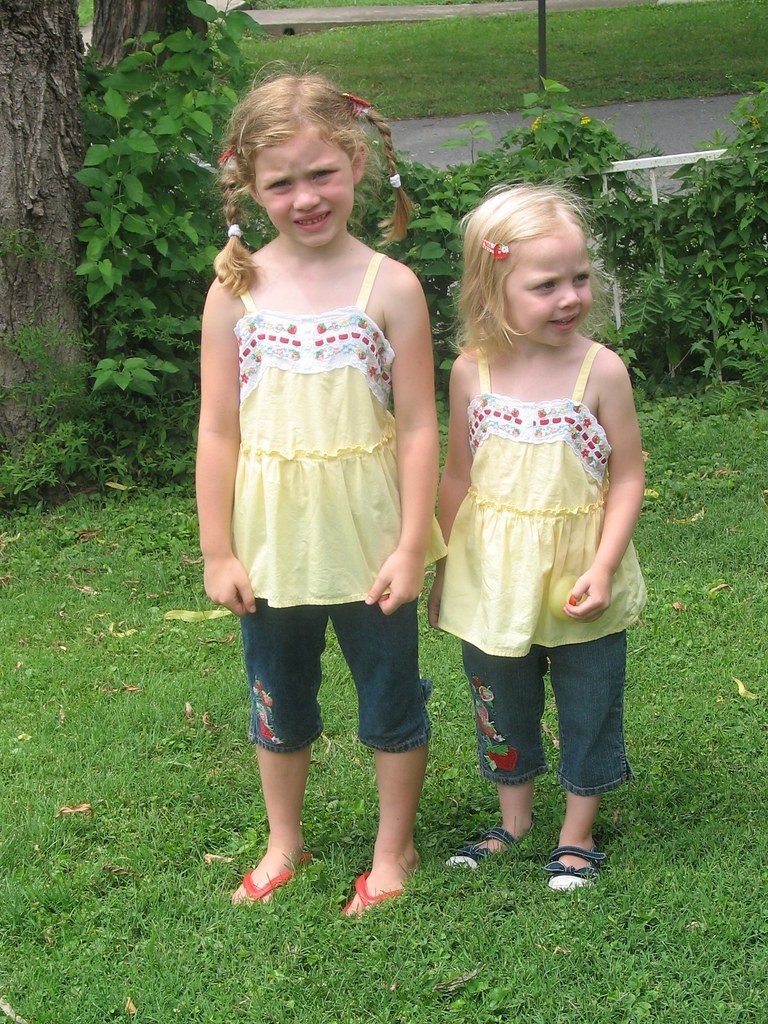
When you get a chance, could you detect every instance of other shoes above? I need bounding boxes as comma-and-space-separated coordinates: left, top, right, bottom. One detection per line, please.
445, 823, 536, 870
544, 841, 605, 894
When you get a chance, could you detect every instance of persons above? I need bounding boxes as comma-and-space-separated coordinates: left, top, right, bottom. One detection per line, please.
427, 181, 648, 892
196, 60, 449, 920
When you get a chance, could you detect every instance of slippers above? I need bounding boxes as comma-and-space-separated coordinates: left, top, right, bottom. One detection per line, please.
338, 870, 409, 923
231, 849, 312, 912
